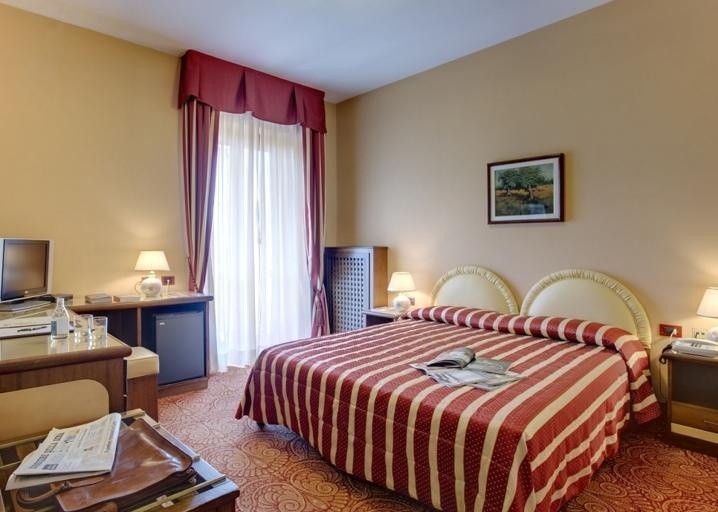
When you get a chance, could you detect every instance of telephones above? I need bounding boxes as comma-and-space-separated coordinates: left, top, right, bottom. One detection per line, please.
671, 337, 718, 358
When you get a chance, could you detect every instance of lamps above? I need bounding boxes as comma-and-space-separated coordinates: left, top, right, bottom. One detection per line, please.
134, 250, 170, 299
387, 272, 416, 312
696, 287, 718, 343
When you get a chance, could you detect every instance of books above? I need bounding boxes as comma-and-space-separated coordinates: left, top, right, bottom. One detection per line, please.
85, 294, 112, 303
114, 295, 139, 302
408, 347, 527, 391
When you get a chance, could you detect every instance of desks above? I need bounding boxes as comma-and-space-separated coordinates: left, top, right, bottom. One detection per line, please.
0, 308, 133, 467
1, 407, 241, 511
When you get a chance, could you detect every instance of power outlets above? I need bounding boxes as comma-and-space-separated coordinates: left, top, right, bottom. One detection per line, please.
659, 324, 682, 338
409, 297, 415, 304
161, 276, 176, 285
692, 327, 707, 340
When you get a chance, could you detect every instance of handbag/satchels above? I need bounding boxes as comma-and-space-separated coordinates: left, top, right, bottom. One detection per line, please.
15, 420, 196, 511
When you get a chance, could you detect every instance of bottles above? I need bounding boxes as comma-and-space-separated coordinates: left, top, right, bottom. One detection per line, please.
50, 296, 69, 338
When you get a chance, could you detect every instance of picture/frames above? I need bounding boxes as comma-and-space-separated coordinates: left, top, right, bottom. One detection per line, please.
487, 153, 565, 225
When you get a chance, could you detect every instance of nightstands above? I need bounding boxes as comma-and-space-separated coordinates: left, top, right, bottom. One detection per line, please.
662, 348, 718, 457
361, 306, 397, 327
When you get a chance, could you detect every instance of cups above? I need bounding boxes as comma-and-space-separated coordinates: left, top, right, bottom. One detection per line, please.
88, 318, 105, 343
75, 314, 91, 339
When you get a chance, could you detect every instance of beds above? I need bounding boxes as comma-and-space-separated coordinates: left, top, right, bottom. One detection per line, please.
235, 264, 663, 511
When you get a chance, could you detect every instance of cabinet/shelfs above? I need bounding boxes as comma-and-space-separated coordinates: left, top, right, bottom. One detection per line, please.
141, 301, 209, 390
74, 307, 139, 346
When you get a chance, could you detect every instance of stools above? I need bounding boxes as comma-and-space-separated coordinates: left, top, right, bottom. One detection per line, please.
123, 345, 160, 422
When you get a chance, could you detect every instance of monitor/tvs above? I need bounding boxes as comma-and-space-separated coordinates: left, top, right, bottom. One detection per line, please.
2, 237, 55, 311
